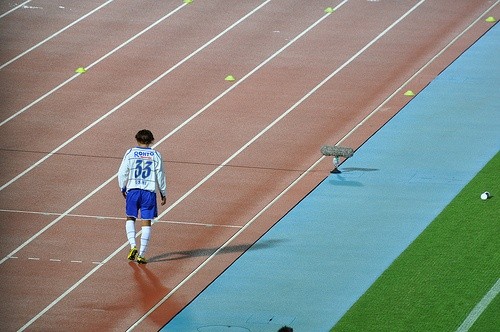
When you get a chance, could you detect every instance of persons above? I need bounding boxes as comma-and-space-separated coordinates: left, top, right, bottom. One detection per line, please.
118, 130, 167, 265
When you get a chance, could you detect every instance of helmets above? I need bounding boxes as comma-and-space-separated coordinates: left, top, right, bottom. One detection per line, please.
135, 129, 154, 141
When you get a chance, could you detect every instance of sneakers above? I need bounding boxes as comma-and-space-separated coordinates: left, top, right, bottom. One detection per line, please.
136, 256, 147, 264
127, 246, 138, 260
